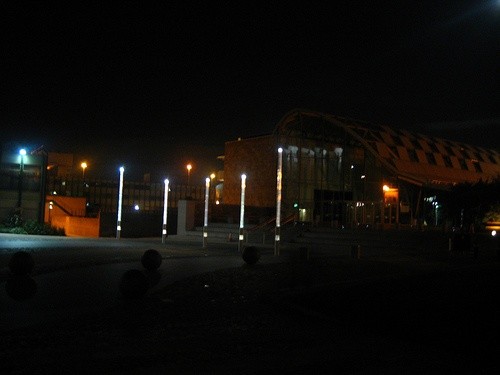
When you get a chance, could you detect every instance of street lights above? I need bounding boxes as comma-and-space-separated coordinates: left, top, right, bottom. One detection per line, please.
187, 164, 192, 199
202, 177, 210, 248
273, 147, 284, 261
116, 167, 125, 239
238, 174, 246, 241
161, 178, 170, 243
210, 173, 216, 205
81, 162, 87, 198
15, 149, 26, 227
